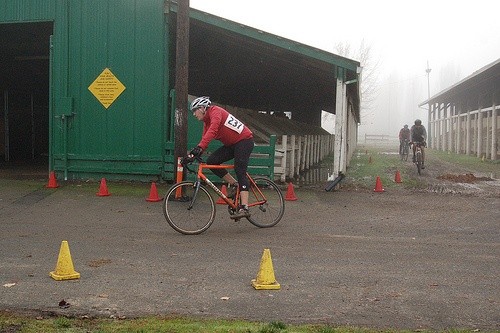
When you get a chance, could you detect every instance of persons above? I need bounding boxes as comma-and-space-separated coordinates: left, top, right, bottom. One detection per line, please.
411, 120, 426, 169
186, 96, 254, 218
399, 125, 411, 158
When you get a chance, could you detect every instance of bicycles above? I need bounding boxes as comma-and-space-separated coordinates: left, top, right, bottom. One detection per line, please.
400, 138, 412, 164
408, 140, 425, 176
163, 154, 286, 236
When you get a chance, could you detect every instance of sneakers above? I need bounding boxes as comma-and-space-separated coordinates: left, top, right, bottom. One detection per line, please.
227, 182, 237, 197
230, 209, 251, 218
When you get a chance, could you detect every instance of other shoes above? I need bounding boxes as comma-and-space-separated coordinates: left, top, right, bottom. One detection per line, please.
422, 166, 425, 169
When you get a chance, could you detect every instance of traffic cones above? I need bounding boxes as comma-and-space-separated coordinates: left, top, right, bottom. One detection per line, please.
282, 182, 298, 201
145, 182, 164, 203
96, 178, 112, 197
45, 172, 60, 188
215, 184, 232, 205
250, 248, 282, 290
48, 241, 81, 281
372, 176, 386, 192
394, 170, 403, 184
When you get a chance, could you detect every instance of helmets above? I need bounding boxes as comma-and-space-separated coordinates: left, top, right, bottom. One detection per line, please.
190, 96, 211, 111
415, 120, 421, 124
